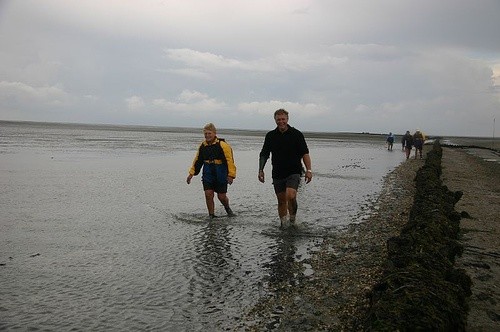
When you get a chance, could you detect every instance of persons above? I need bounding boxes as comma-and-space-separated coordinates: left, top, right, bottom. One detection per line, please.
386, 128, 425, 159
258, 109, 313, 229
186, 123, 236, 220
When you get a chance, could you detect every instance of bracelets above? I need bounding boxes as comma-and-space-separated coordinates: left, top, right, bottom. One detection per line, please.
306, 170, 312, 172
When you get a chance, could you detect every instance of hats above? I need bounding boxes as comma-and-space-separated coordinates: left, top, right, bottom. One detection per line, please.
416, 128, 420, 132
